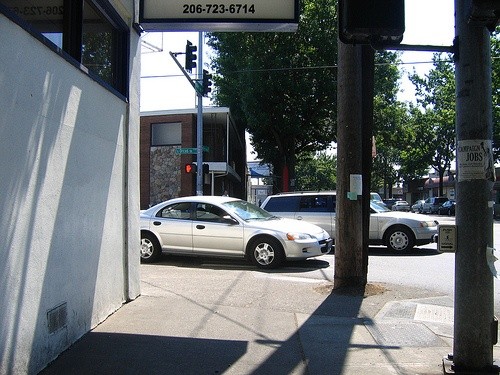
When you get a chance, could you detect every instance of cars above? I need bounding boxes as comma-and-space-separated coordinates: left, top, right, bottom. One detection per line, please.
411, 200, 426, 213
390, 201, 410, 212
140, 195, 333, 270
440, 199, 456, 216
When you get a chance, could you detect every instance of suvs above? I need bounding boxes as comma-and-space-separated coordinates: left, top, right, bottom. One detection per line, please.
259, 191, 442, 257
424, 197, 448, 214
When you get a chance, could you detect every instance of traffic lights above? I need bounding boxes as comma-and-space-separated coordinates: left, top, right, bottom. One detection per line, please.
185, 39, 198, 71
185, 162, 195, 175
202, 68, 213, 98
